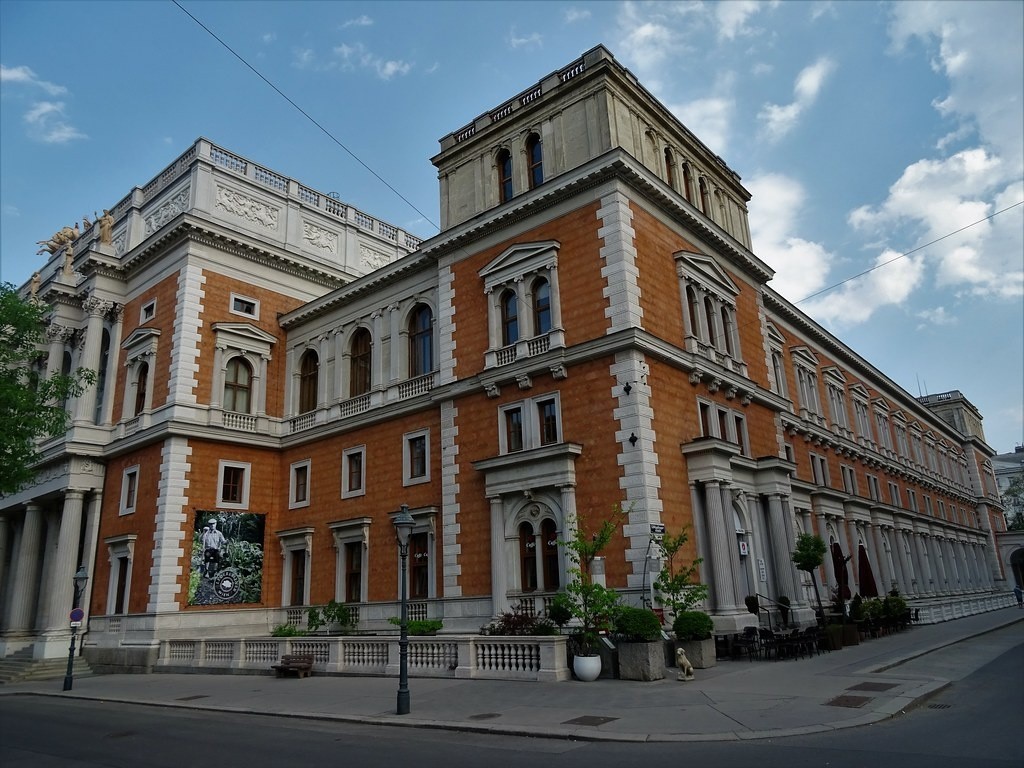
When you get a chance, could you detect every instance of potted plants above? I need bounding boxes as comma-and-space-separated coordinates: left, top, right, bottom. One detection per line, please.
850, 594, 907, 638
613, 609, 666, 682
553, 565, 619, 682
672, 610, 716, 670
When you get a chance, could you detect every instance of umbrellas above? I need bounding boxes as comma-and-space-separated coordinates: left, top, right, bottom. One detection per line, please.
833, 541, 852, 603
857, 541, 878, 601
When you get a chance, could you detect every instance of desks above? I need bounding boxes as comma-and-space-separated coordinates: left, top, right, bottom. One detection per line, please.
775, 628, 805, 657
714, 630, 741, 660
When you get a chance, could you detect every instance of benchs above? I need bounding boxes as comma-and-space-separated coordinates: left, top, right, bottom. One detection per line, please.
270, 654, 314, 679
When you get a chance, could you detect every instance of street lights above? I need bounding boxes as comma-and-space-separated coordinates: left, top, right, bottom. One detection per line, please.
63, 566, 89, 691
393, 504, 415, 714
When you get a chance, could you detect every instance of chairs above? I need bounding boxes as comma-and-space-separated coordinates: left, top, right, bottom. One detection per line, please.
735, 626, 833, 661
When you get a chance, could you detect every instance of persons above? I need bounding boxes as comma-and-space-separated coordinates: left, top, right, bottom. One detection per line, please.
95, 208, 114, 244
65, 244, 74, 268
1014, 585, 1023, 607
199, 519, 229, 582
32, 272, 42, 292
